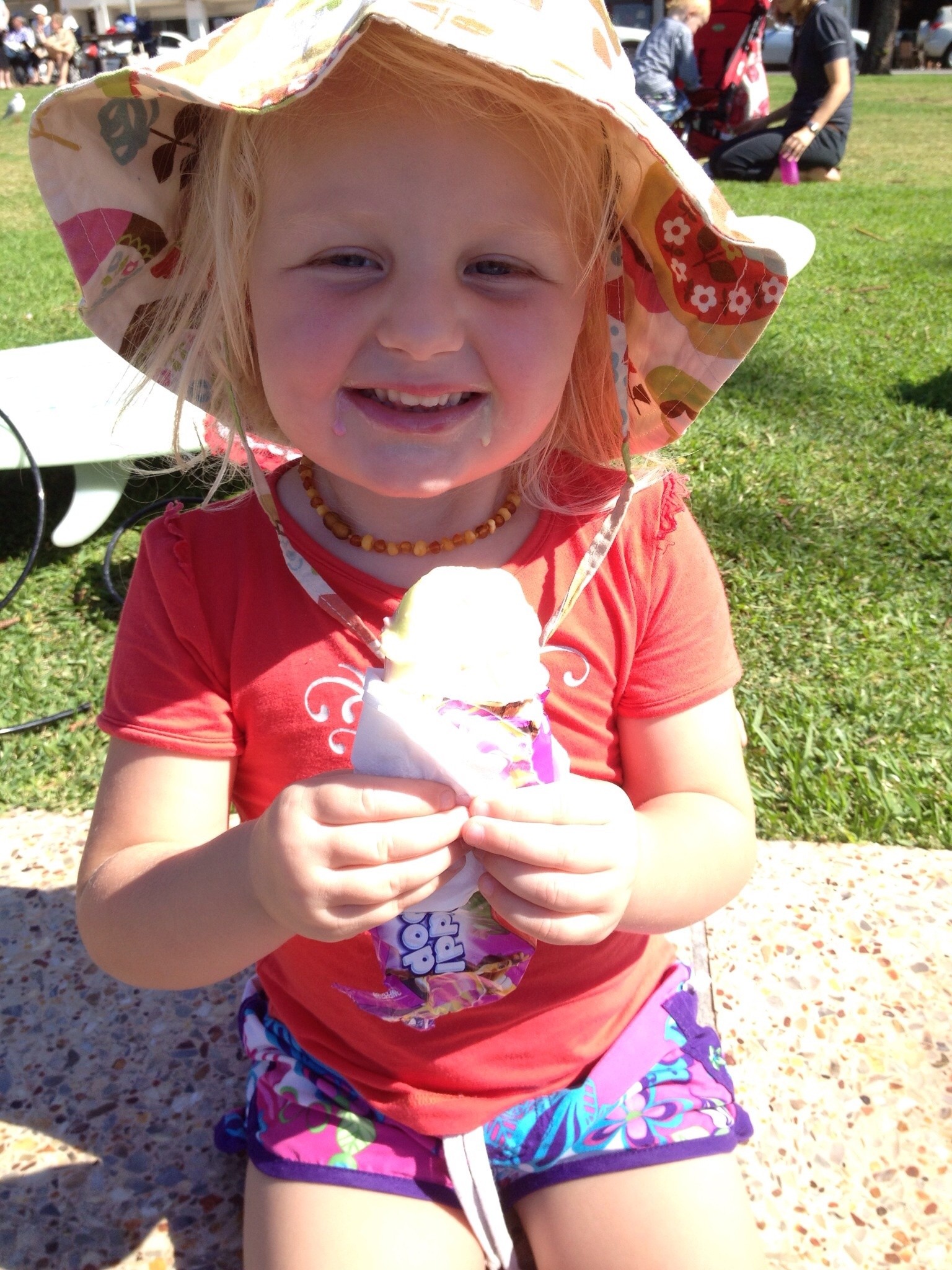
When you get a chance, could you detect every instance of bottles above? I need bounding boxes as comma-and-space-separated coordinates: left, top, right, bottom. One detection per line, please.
779, 142, 800, 185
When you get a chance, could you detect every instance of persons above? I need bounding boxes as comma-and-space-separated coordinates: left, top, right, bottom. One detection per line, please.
634, 0, 712, 147
0, 0, 81, 89
34, 0, 786, 1270
708, 0, 856, 183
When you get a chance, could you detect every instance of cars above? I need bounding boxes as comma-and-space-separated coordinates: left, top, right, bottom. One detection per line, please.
76, 30, 193, 77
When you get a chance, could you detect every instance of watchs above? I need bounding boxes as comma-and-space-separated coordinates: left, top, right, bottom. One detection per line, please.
805, 122, 820, 136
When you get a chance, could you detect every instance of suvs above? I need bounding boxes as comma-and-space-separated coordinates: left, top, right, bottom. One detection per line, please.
916, 5, 952, 70
603, 0, 871, 72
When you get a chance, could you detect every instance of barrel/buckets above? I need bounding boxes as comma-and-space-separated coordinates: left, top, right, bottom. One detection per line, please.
100, 58, 121, 72
78, 59, 96, 79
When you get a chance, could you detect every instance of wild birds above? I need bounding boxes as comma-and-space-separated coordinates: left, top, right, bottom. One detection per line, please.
1, 92, 25, 123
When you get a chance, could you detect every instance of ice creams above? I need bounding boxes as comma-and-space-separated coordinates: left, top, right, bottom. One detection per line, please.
380, 556, 550, 724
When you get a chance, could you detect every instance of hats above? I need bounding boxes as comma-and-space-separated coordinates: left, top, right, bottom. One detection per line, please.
27, 0, 816, 456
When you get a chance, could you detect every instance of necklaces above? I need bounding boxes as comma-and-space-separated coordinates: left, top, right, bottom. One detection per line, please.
298, 454, 527, 555
